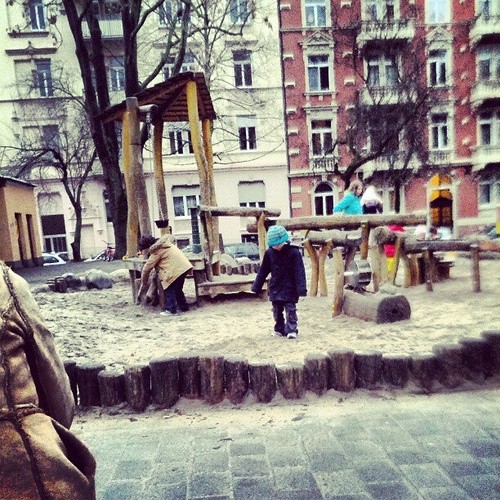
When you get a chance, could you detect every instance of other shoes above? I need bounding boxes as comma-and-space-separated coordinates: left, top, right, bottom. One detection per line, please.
160, 310, 174, 315
274, 332, 282, 337
287, 331, 297, 339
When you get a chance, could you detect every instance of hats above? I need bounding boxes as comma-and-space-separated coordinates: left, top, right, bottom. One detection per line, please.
266, 225, 289, 246
139, 236, 155, 250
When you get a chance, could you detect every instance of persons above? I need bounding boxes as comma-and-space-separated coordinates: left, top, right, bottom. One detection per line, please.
333, 180, 443, 285
137, 235, 194, 316
250, 225, 307, 340
0, 256, 96, 500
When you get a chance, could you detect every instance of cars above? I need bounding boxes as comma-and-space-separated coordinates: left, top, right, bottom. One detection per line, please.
83, 249, 115, 263
42, 252, 69, 267
180, 243, 259, 260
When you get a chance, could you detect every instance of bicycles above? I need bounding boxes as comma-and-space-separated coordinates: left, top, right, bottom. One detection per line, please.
102, 240, 114, 261
